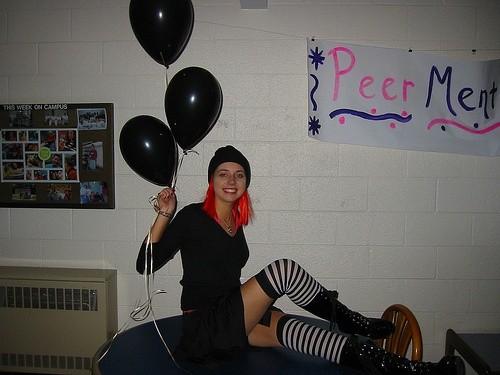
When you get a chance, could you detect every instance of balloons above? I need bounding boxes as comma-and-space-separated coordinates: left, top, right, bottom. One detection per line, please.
119, 114, 179, 188
165, 65, 223, 152
129, 0, 195, 67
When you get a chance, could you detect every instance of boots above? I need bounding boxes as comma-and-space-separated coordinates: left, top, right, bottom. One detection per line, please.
339, 335, 465, 375
301, 286, 396, 338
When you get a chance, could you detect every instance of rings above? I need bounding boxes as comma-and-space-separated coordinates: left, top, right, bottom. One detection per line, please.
158, 193, 160, 196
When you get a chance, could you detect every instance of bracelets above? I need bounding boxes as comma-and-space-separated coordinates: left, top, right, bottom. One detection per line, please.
157, 209, 173, 219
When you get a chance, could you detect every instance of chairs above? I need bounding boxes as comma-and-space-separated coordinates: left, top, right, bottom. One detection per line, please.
376, 304, 423, 362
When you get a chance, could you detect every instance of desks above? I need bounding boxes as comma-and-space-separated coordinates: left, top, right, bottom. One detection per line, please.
93, 315, 409, 375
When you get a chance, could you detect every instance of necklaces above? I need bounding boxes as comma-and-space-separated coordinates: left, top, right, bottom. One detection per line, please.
217, 211, 233, 233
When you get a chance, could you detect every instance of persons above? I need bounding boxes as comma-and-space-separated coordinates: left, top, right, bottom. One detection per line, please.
135, 145, 466, 375
0, 104, 113, 206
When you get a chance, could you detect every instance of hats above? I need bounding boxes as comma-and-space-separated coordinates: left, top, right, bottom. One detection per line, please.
208, 145, 251, 188
